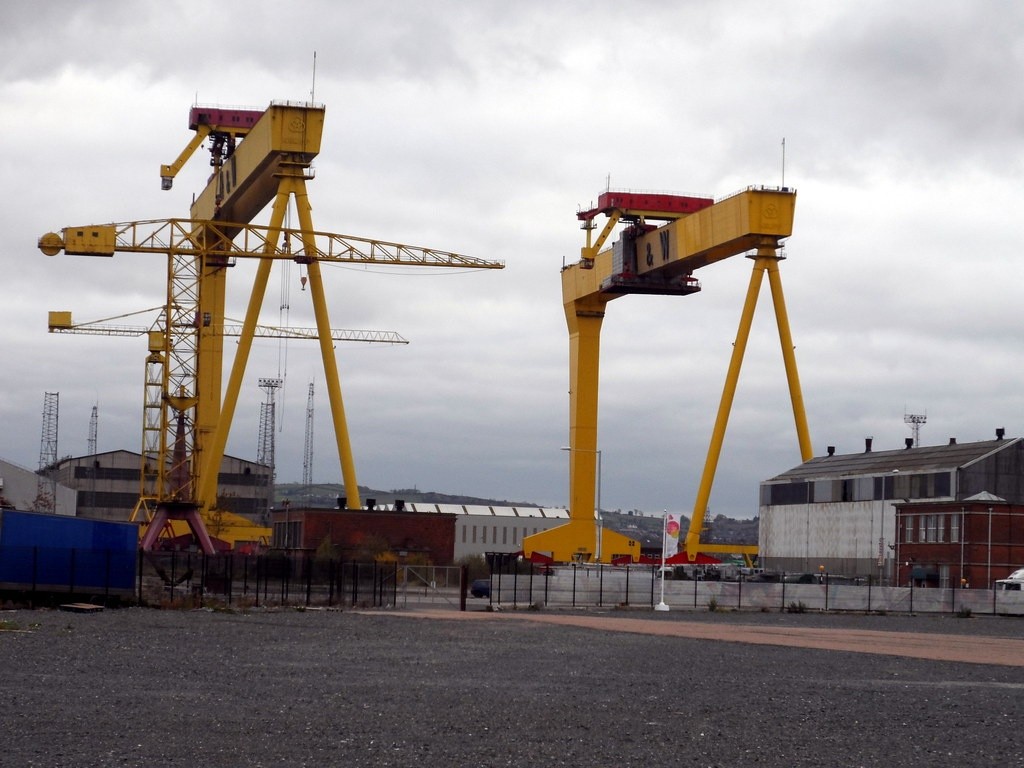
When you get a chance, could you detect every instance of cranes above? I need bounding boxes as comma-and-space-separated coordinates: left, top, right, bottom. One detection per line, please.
39, 101, 503, 548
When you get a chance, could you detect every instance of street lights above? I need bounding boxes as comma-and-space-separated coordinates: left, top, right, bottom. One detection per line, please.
558, 445, 602, 562
876, 469, 898, 584
